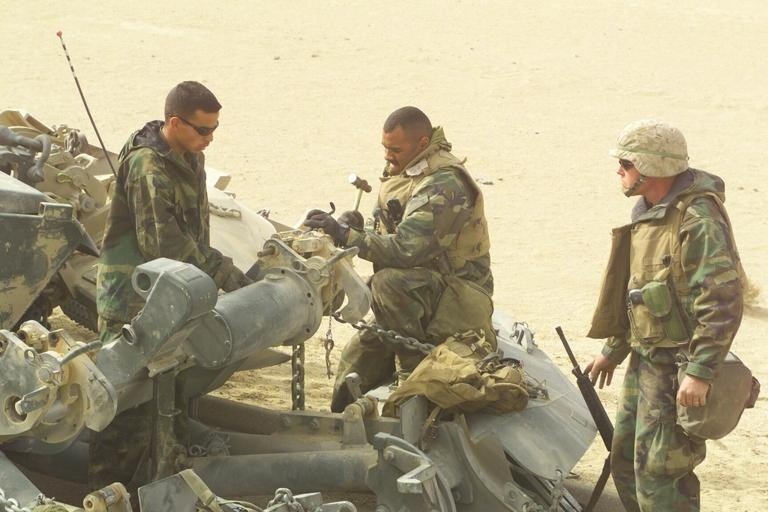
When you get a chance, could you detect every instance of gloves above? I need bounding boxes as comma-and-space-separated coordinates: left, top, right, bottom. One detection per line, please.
302, 210, 349, 243
217, 253, 255, 293
339, 208, 364, 231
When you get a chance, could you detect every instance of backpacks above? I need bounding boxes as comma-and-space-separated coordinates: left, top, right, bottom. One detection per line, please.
386, 330, 533, 427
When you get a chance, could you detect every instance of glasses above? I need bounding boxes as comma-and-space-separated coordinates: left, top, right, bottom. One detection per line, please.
169, 115, 221, 135
617, 156, 634, 171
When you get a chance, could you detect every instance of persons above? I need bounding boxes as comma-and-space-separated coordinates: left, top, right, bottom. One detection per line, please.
93, 80, 256, 349
582, 119, 749, 512
299, 106, 496, 414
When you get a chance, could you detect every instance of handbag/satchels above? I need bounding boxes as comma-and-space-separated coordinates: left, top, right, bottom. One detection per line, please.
677, 349, 754, 444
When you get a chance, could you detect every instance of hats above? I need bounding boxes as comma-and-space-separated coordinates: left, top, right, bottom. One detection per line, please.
608, 117, 689, 178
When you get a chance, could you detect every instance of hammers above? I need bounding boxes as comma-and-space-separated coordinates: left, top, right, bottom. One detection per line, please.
349, 174, 372, 211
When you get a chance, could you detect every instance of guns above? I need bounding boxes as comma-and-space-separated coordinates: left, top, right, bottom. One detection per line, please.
557, 326, 614, 512
